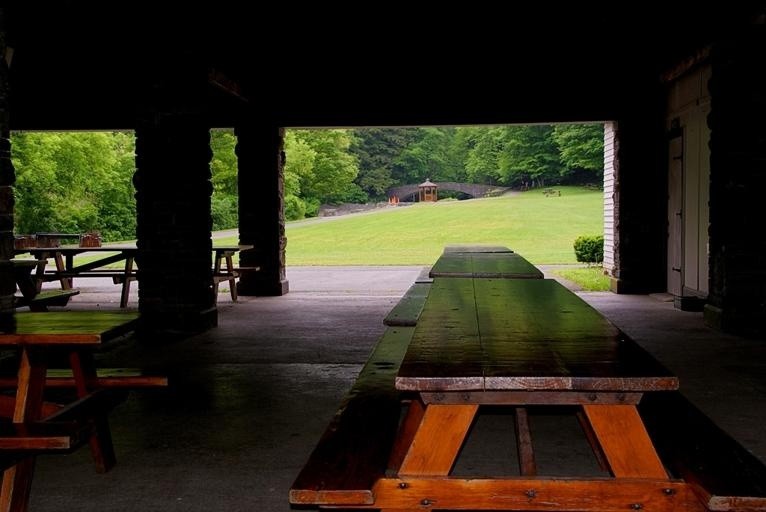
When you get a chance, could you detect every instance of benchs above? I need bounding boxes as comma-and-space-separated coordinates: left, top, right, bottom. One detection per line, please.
635, 391, 766, 512
0, 267, 260, 512
289, 269, 435, 512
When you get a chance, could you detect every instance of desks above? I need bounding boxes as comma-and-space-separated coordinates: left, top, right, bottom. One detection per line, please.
0, 260, 49, 311
14, 233, 81, 247
30, 243, 254, 308
428, 245, 545, 277
375, 278, 680, 512
